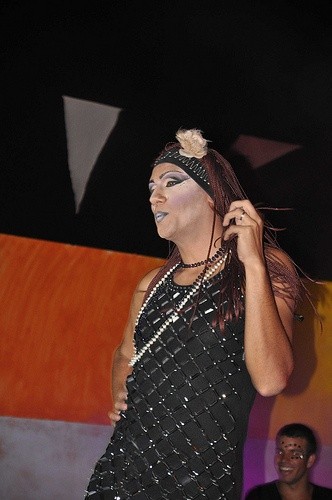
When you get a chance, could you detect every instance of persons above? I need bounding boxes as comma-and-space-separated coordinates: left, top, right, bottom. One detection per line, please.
83, 127, 315, 500
244, 423, 332, 500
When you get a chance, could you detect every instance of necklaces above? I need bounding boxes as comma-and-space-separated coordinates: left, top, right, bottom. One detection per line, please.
129, 243, 231, 370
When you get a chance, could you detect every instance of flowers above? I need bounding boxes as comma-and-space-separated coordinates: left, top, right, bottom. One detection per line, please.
176, 128, 210, 158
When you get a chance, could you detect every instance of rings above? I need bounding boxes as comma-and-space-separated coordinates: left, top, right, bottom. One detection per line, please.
239, 211, 245, 222
